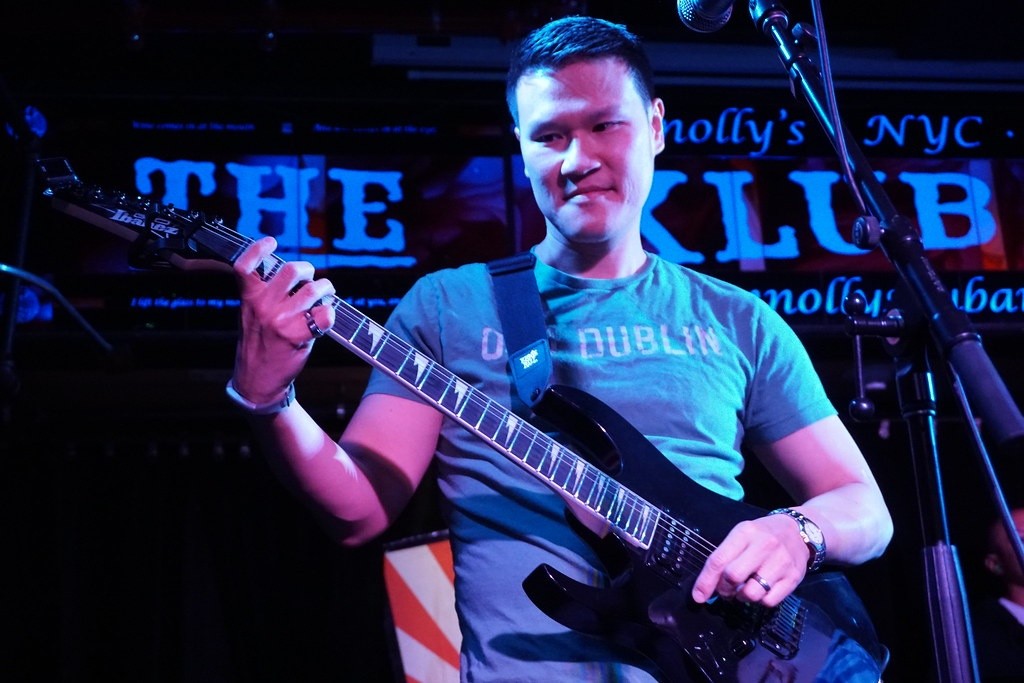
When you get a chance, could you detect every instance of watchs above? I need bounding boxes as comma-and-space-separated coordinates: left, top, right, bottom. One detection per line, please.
768, 508, 827, 572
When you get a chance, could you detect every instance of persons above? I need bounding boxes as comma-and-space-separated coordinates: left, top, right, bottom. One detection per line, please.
222, 15, 896, 682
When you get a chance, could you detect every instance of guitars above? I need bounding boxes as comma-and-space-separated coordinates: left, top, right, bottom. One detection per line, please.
34, 169, 891, 683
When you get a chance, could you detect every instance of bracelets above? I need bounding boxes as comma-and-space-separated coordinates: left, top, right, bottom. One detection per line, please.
225, 377, 297, 415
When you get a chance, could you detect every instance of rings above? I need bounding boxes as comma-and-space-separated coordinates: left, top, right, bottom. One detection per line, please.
752, 573, 771, 592
305, 311, 324, 338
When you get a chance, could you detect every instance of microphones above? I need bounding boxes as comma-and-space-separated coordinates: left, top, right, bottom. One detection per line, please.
677, 0, 733, 33
